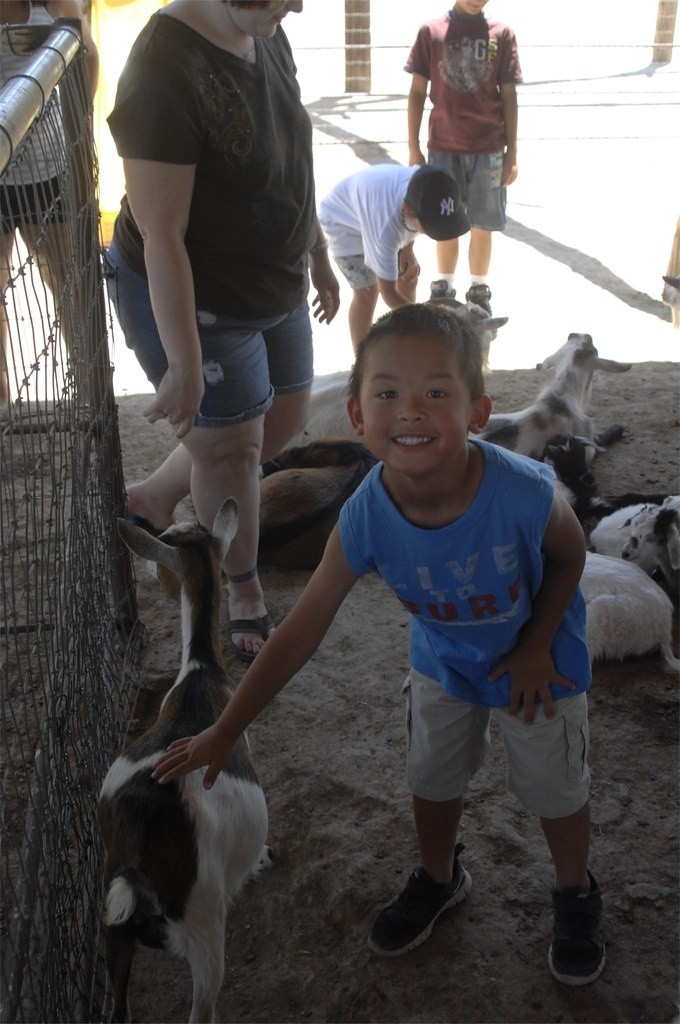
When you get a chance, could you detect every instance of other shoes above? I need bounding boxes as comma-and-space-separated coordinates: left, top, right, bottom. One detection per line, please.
465, 284, 492, 315
429, 280, 456, 300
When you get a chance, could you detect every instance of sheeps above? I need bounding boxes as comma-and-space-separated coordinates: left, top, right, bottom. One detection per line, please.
98, 497, 269, 1024
259, 280, 680, 672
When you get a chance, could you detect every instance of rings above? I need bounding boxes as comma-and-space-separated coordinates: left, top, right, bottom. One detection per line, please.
160, 409, 168, 416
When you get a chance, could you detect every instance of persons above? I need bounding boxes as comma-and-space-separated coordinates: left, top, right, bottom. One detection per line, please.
320, 163, 469, 358
150, 303, 604, 986
0, 0, 98, 420
402, 0, 525, 316
102, 0, 340, 664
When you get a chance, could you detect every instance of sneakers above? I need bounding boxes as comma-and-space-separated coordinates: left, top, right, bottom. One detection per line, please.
368, 842, 471, 957
547, 868, 606, 985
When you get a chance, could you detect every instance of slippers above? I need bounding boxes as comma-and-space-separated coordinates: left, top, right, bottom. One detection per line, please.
126, 513, 162, 537
225, 594, 275, 660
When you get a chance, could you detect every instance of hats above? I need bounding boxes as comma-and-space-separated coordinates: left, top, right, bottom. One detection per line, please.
408, 164, 471, 241
